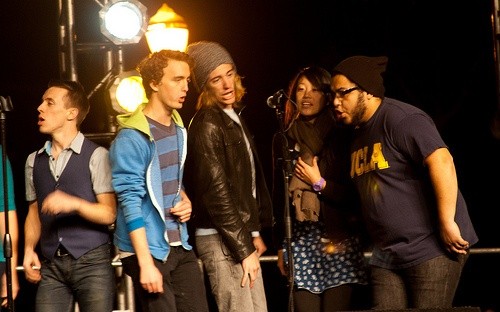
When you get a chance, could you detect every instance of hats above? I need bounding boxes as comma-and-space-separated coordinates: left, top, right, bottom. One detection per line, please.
333, 55, 388, 100
186, 40, 234, 92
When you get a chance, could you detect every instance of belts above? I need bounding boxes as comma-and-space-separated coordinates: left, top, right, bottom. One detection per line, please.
54, 248, 69, 258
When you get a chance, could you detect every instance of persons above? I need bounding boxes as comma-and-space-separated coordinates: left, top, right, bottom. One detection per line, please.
271, 56, 479, 312
0, 96, 19, 308
109, 49, 197, 312
21, 81, 117, 312
186, 41, 268, 312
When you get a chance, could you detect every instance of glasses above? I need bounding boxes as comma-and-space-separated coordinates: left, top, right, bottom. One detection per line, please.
327, 86, 361, 99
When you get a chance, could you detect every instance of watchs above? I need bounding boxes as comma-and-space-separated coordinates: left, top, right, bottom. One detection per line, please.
312, 177, 324, 191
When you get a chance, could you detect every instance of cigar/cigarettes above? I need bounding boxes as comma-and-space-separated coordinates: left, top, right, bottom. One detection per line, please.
15, 266, 40, 270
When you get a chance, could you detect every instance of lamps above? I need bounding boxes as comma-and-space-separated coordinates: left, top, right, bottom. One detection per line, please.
145, 2, 190, 56
98, 0, 146, 46
106, 69, 153, 116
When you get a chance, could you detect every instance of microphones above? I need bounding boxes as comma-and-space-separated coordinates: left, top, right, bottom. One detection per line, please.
266, 90, 284, 109
